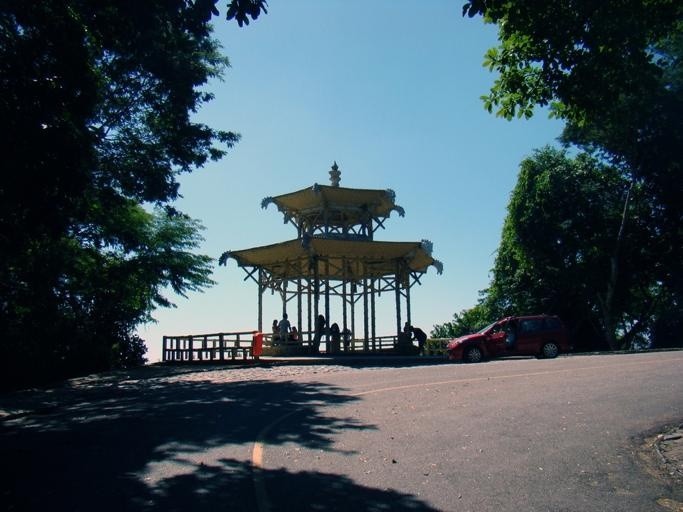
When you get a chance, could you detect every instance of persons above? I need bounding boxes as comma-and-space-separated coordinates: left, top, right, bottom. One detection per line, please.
410, 326, 428, 357
271, 315, 298, 342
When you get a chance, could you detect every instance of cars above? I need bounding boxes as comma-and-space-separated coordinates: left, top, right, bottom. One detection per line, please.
446, 313, 568, 363
